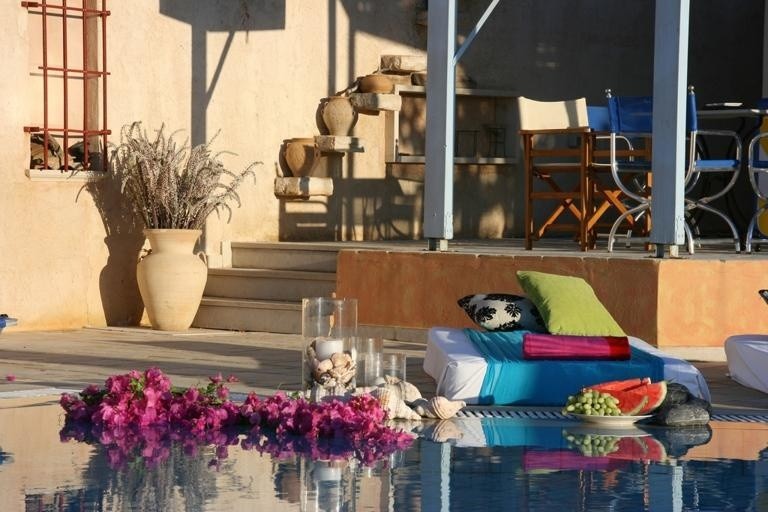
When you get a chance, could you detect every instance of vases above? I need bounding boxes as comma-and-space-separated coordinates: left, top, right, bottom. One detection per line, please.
136, 228, 208, 329
321, 96, 357, 136
359, 75, 395, 93
284, 139, 322, 177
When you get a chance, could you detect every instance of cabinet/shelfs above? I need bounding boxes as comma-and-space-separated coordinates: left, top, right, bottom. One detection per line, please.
385, 84, 520, 166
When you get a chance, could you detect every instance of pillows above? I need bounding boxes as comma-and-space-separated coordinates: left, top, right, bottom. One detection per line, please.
523, 449, 634, 472
515, 270, 628, 337
516, 469, 572, 475
457, 293, 546, 331
524, 334, 632, 361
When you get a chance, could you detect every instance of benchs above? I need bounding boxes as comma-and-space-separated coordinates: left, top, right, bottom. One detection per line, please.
449, 418, 693, 456
422, 326, 711, 406
724, 335, 768, 396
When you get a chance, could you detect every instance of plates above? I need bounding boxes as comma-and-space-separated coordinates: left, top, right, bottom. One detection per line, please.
574, 412, 654, 429
705, 101, 742, 108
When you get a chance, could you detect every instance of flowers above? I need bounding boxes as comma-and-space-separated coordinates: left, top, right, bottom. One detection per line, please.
60, 365, 386, 437
59, 427, 416, 472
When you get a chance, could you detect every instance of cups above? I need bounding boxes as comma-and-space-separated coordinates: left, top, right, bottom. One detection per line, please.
300, 296, 358, 388
349, 335, 406, 387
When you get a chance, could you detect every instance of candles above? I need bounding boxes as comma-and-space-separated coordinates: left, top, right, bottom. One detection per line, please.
315, 326, 343, 362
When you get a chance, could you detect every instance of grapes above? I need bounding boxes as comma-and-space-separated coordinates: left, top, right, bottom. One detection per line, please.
561, 391, 621, 417
562, 430, 621, 458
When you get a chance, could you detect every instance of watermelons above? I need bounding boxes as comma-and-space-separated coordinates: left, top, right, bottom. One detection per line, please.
582, 376, 668, 416
604, 435, 669, 464
